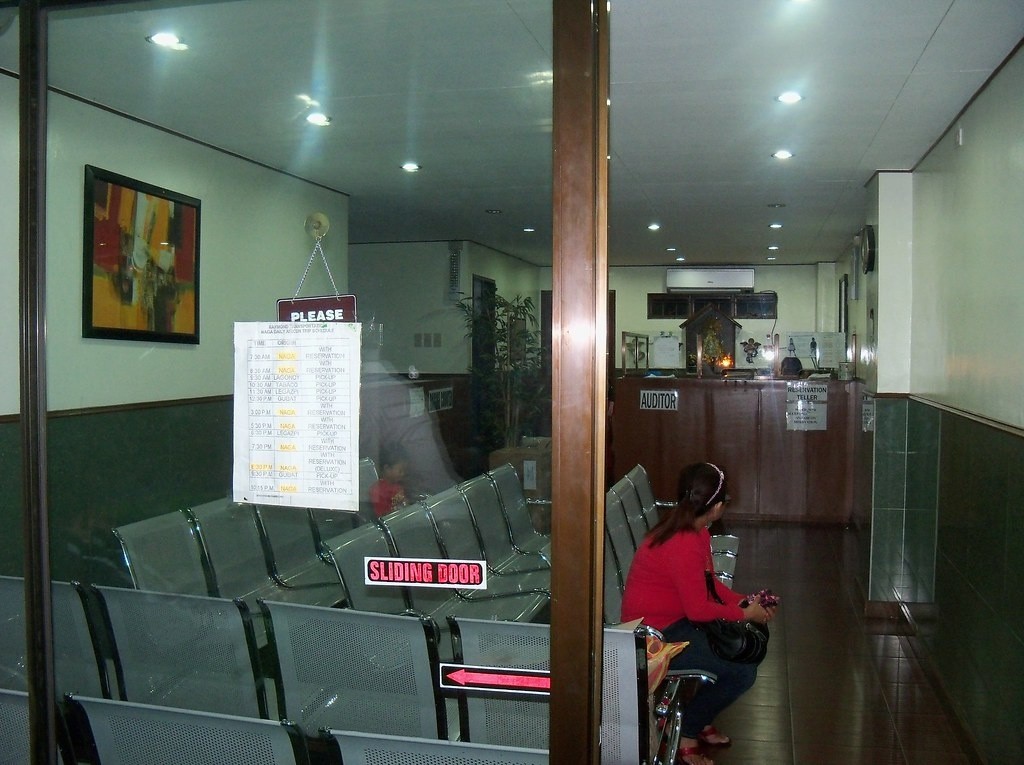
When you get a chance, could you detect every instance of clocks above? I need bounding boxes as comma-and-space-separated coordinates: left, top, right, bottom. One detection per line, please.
860, 225, 874, 274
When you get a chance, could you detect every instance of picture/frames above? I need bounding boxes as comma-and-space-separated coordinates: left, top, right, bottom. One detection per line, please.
82, 163, 201, 344
838, 274, 848, 335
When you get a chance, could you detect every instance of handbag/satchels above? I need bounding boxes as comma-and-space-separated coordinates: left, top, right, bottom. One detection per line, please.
703, 569, 769, 664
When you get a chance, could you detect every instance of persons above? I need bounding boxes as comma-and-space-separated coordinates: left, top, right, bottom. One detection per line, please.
357, 341, 459, 506
369, 441, 412, 517
619, 461, 780, 765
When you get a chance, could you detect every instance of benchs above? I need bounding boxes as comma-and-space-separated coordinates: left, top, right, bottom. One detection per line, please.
0, 457, 739, 765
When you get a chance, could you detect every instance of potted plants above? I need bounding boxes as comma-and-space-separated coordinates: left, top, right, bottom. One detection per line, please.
457, 290, 552, 529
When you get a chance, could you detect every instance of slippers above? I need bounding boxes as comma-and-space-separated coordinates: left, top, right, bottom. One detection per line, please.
663, 739, 713, 765
699, 723, 732, 749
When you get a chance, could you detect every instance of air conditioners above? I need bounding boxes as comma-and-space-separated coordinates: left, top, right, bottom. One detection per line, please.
666, 268, 754, 294
